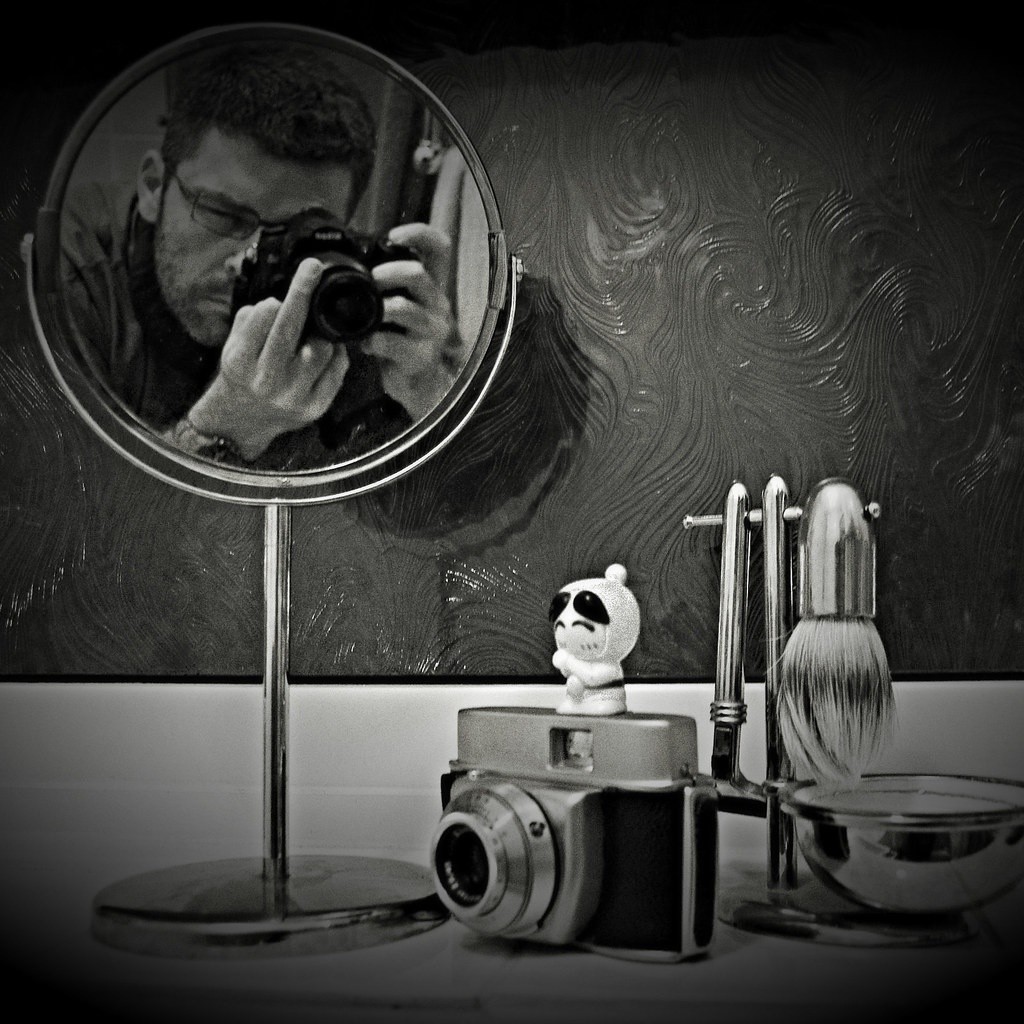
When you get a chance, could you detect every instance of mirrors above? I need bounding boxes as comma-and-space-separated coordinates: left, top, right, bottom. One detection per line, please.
9, 18, 534, 962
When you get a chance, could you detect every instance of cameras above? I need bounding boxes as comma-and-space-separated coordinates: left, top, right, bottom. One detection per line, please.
428, 706, 719, 960
230, 207, 418, 352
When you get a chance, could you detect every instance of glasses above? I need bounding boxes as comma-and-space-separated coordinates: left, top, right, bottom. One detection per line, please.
171, 169, 278, 241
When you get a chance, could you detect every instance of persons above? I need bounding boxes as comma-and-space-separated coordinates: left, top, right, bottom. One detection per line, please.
62, 27, 472, 473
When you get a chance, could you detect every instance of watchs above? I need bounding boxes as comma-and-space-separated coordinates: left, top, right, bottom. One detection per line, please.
167, 415, 251, 468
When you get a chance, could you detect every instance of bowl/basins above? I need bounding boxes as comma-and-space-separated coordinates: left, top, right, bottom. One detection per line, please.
777, 775, 1024, 912
